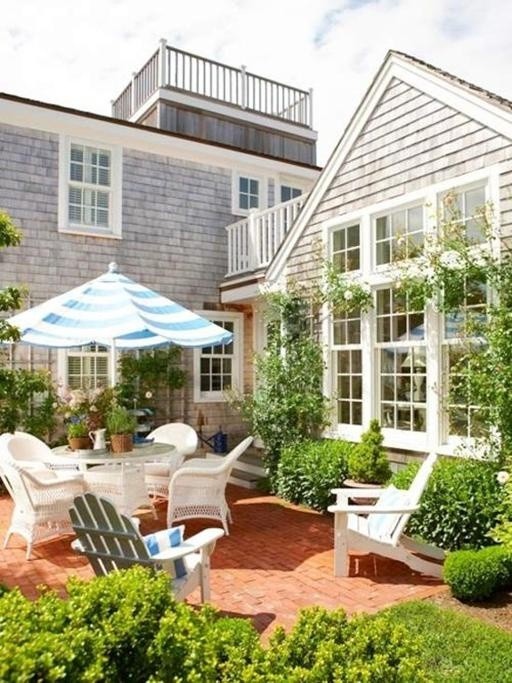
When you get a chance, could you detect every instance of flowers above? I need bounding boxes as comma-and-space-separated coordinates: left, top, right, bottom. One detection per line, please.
56, 377, 113, 429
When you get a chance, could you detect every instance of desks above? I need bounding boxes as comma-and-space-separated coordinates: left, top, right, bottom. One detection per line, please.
50, 441, 176, 521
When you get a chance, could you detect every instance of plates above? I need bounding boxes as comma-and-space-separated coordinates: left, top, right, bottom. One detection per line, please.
65, 444, 108, 454
132, 439, 154, 446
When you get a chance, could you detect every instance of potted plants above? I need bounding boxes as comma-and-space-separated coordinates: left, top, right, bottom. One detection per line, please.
343, 418, 392, 505
105, 398, 134, 452
68, 421, 91, 448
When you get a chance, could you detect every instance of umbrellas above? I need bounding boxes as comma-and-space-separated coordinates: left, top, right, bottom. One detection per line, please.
1, 261, 237, 402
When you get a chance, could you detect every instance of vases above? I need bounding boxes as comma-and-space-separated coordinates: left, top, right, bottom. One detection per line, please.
343, 477, 384, 505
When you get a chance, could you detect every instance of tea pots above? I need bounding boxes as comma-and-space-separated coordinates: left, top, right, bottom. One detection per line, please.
89, 429, 106, 449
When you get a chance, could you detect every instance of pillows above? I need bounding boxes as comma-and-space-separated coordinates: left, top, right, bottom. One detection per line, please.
140, 524, 188, 580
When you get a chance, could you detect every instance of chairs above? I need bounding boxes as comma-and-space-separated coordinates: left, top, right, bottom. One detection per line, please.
145, 423, 199, 501
0, 431, 50, 465
0, 457, 91, 561
167, 435, 253, 535
71, 494, 225, 606
327, 450, 445, 578
